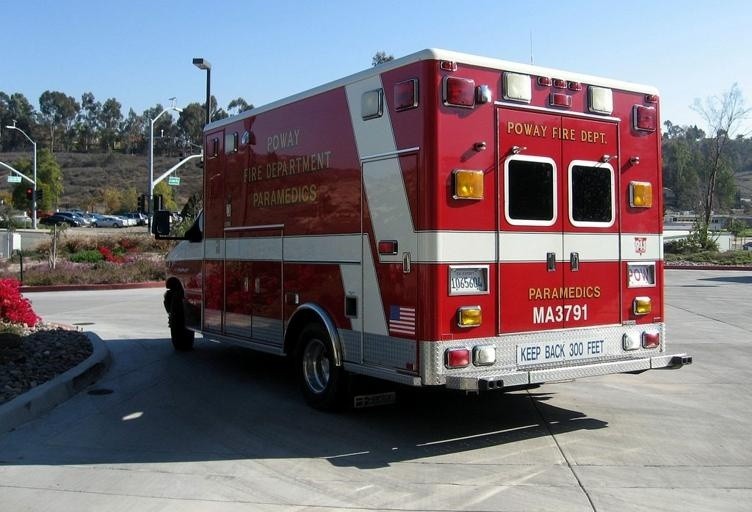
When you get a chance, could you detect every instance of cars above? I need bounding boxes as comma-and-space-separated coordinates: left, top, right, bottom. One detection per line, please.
39, 207, 149, 229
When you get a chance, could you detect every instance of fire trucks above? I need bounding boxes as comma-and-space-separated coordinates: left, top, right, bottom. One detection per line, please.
163, 44, 695, 413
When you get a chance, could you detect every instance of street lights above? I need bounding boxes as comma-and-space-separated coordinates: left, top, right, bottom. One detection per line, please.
5, 119, 37, 229
149, 96, 186, 234
191, 57, 212, 125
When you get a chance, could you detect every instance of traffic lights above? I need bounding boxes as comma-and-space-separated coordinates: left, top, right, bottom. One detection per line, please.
26, 187, 33, 201
137, 194, 145, 214
